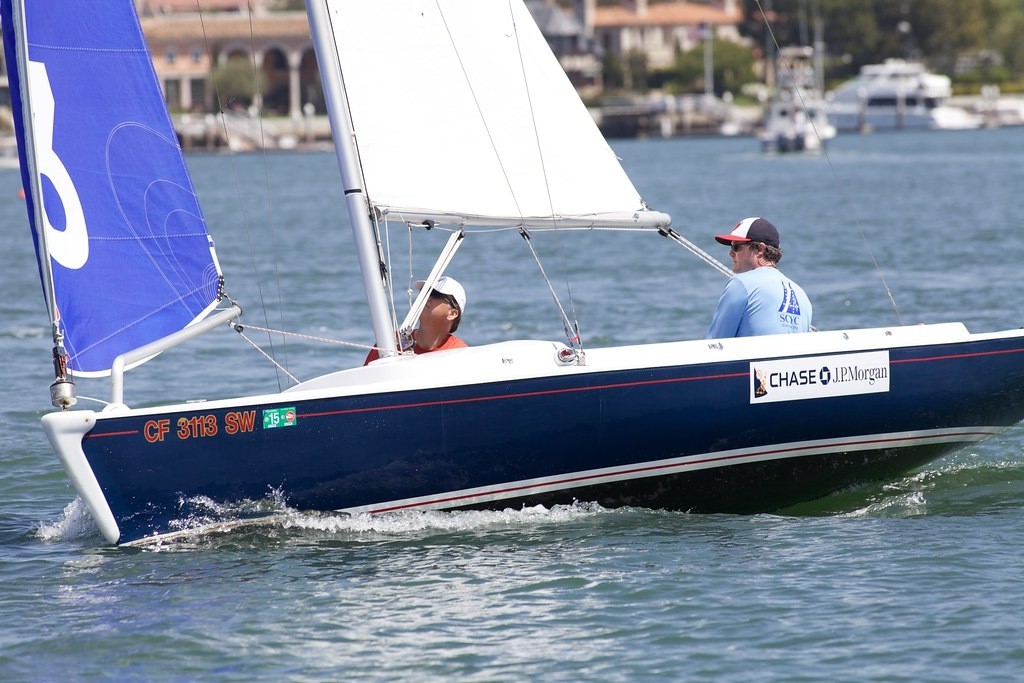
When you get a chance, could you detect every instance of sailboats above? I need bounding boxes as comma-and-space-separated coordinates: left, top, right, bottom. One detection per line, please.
1, 1, 1024, 550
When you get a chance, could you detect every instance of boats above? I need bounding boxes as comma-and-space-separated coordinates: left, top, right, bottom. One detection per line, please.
821, 56, 983, 129
756, 43, 840, 154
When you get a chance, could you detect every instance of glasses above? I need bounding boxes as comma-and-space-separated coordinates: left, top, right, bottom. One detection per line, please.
731, 242, 751, 250
431, 288, 452, 305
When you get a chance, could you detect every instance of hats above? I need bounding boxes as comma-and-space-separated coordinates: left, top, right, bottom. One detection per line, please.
715, 217, 779, 249
413, 275, 466, 316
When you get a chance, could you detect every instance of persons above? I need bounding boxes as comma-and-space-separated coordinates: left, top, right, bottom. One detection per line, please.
706, 216, 813, 339
364, 276, 469, 365
